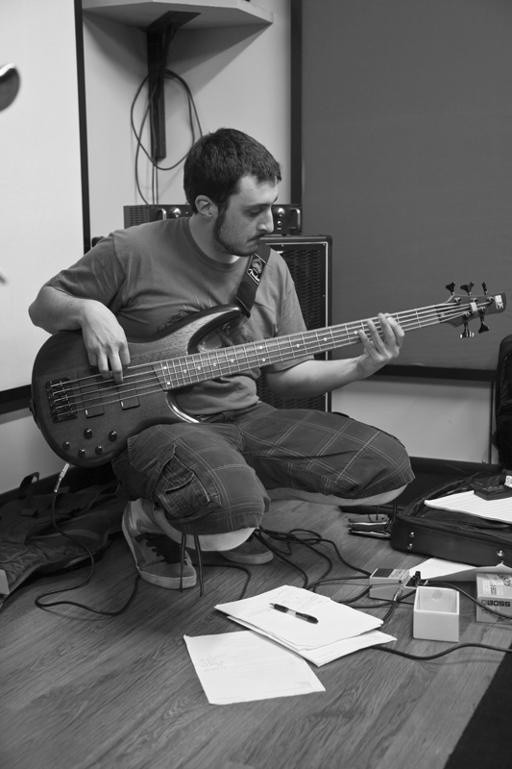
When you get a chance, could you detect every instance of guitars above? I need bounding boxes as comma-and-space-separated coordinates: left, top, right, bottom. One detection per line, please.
31, 282, 508, 469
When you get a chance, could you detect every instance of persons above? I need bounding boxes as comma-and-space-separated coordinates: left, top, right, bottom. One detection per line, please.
27, 127, 414, 591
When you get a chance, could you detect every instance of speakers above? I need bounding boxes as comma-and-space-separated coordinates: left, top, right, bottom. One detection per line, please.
258, 233, 332, 414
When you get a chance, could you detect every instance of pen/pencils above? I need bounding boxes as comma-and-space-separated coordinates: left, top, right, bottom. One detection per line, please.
269, 603, 318, 624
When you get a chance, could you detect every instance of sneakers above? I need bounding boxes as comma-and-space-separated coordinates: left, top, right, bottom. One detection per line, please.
217, 530, 274, 566
121, 498, 199, 593
